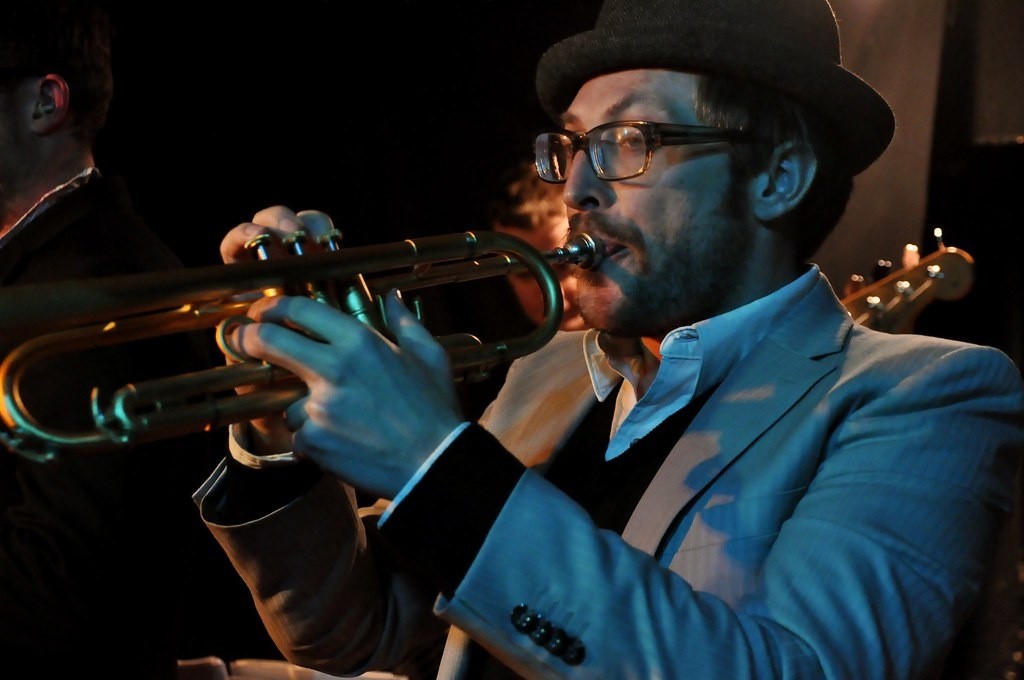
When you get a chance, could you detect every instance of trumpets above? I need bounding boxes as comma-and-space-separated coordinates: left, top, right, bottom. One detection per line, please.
0, 225, 605, 471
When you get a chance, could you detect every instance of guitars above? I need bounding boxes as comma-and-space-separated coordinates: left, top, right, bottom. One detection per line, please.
838, 225, 976, 332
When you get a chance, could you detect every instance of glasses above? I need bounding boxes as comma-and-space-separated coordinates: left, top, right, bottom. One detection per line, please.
533, 124, 778, 185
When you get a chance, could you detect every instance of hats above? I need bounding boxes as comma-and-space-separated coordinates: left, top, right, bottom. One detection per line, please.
534, 0, 894, 176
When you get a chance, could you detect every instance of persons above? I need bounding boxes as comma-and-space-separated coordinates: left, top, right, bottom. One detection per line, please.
494, 160, 588, 332
0, 1, 212, 680
192, 0, 1024, 680
